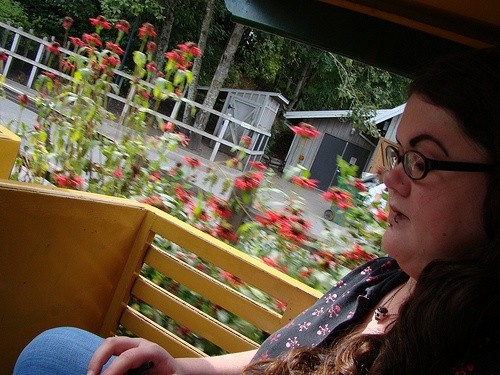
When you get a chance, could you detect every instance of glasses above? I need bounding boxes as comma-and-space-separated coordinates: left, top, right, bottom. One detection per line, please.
384, 145, 488, 180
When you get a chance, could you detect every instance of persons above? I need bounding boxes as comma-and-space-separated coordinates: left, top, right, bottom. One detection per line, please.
14, 55, 500, 375
